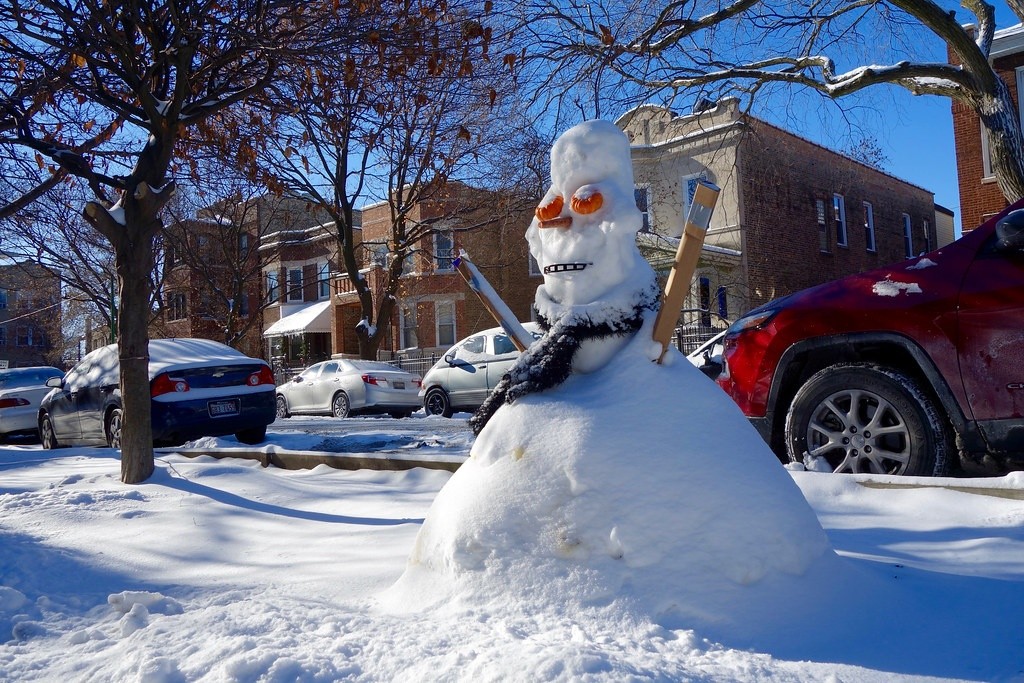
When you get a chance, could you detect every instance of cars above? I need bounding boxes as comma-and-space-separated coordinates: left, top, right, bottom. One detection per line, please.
0, 367, 66, 446
685, 331, 727, 380
420, 321, 546, 419
37, 338, 277, 450
276, 359, 424, 418
715, 197, 1024, 476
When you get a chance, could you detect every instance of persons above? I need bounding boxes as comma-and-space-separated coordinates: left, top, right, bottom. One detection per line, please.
321, 350, 328, 360
